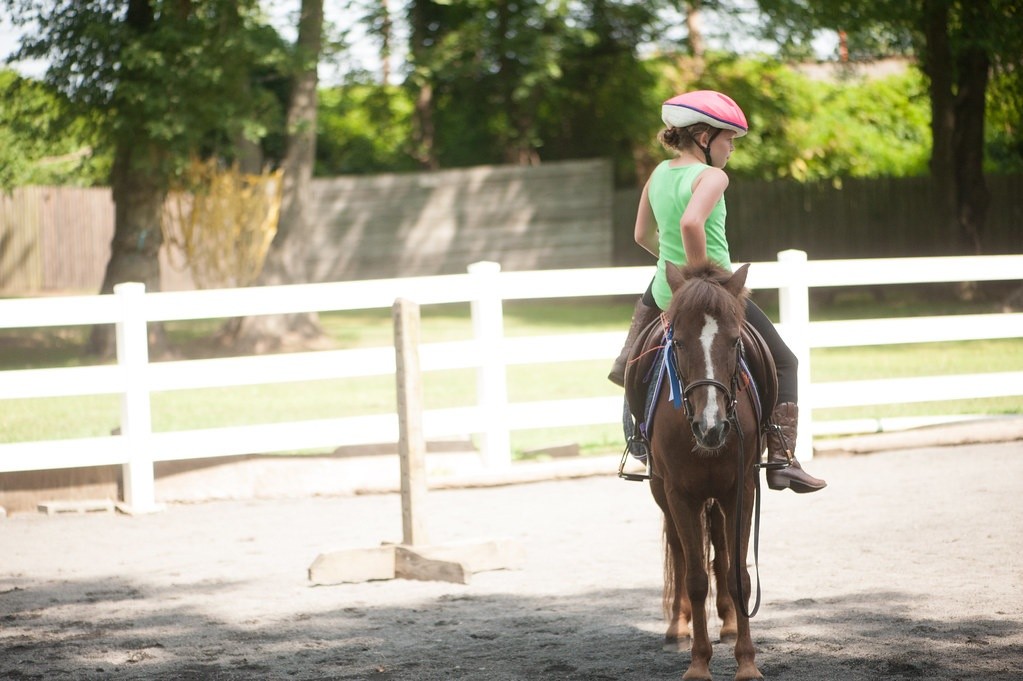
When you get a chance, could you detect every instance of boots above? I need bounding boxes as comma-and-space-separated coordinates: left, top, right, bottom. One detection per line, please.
608, 297, 662, 388
765, 401, 827, 493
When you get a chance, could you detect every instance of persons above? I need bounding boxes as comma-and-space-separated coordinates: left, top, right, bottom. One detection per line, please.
608, 90, 827, 494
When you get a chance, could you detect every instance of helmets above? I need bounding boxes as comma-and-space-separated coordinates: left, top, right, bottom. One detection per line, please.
661, 90, 748, 139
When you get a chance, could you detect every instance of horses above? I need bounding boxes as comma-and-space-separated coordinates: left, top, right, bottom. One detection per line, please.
625, 260, 780, 681
